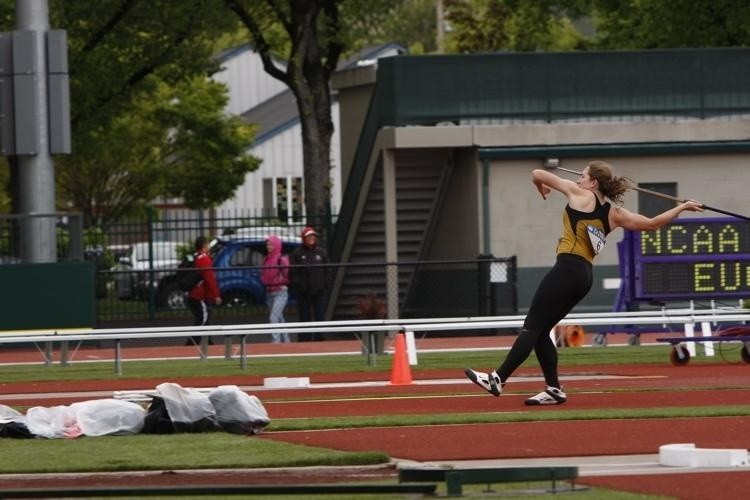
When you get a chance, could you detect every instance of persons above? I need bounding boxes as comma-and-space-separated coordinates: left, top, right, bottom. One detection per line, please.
112, 257, 139, 301
462, 159, 706, 409
287, 226, 333, 342
259, 235, 292, 343
348, 288, 388, 340
178, 237, 223, 347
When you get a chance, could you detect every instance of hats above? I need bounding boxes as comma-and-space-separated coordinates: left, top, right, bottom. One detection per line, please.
301, 227, 318, 237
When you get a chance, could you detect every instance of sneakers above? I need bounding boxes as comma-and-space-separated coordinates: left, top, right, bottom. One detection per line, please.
465, 367, 505, 396
524, 385, 567, 406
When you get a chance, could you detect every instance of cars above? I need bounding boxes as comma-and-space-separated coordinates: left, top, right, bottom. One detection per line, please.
84, 225, 304, 310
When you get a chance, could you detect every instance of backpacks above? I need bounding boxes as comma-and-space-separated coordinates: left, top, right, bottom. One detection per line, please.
176, 251, 204, 292
